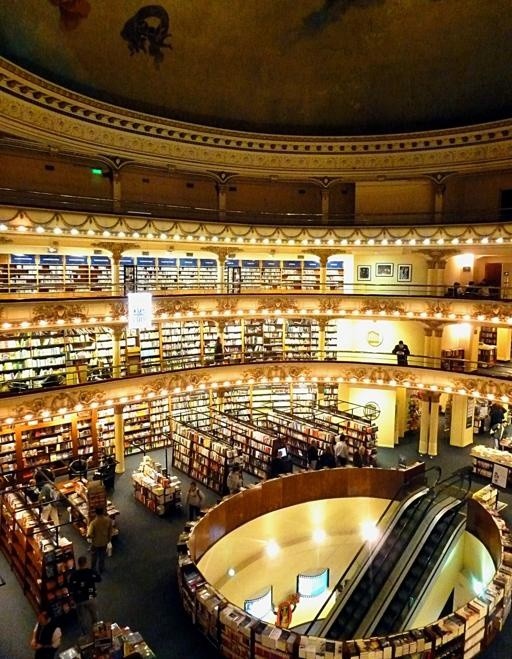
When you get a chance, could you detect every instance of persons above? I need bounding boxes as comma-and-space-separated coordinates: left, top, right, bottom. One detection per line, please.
86, 504, 112, 574
392, 341, 410, 366
25, 478, 61, 532
452, 278, 490, 296
213, 337, 224, 365
68, 556, 102, 635
226, 465, 242, 495
185, 481, 205, 521
30, 610, 63, 659
307, 435, 366, 469
488, 402, 508, 450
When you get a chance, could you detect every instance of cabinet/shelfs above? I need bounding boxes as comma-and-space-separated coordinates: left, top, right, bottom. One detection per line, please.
1, 382, 512, 659
2, 246, 344, 399
